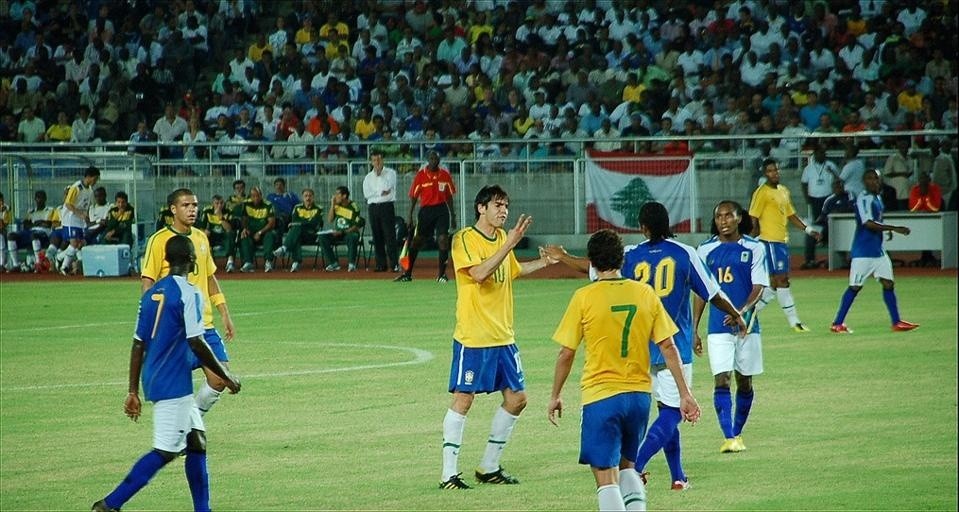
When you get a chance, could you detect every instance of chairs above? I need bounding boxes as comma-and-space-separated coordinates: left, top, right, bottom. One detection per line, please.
297, 215, 405, 271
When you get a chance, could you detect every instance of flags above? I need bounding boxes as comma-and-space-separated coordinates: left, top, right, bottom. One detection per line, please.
582, 149, 703, 234
398, 240, 410, 272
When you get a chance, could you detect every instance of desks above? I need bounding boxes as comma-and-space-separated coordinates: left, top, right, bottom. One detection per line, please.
827, 210, 957, 270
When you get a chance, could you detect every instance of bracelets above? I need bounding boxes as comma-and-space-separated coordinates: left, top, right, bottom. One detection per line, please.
128, 392, 139, 394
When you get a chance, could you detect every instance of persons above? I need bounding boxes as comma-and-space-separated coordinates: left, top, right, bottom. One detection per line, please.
548, 229, 701, 512
746, 157, 821, 331
435, 184, 564, 489
363, 154, 399, 275
1, 0, 959, 176
540, 200, 749, 492
699, 198, 771, 453
392, 151, 455, 284
830, 166, 917, 336
89, 239, 242, 511
799, 176, 959, 271
140, 187, 235, 433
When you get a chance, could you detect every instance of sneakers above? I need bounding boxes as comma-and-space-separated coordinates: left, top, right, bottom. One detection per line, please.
375, 266, 388, 272
91, 498, 121, 511
438, 472, 474, 490
892, 320, 920, 332
736, 435, 746, 451
391, 265, 399, 272
671, 477, 692, 490
637, 471, 651, 487
830, 322, 852, 334
393, 273, 413, 282
791, 322, 809, 332
721, 438, 740, 451
435, 275, 448, 282
475, 466, 519, 485
